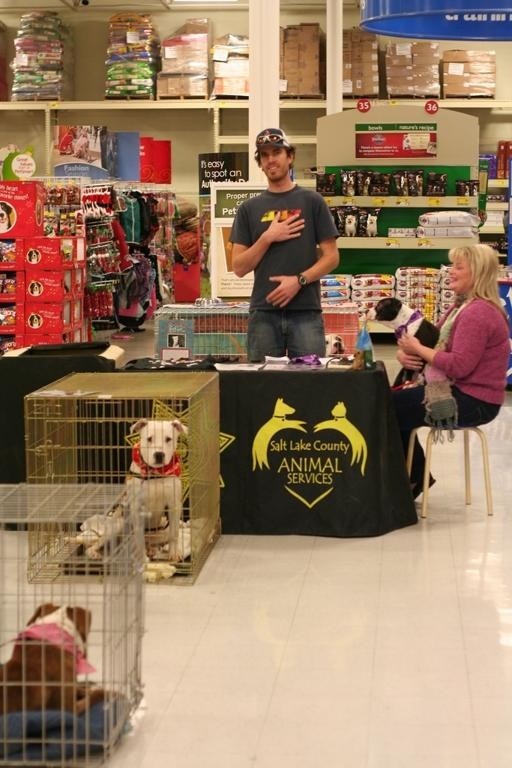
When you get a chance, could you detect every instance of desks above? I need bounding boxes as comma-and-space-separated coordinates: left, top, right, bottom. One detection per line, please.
0, 340, 118, 485
120, 355, 418, 538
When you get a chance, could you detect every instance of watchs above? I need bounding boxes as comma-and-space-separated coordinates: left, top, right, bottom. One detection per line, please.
296, 274, 307, 287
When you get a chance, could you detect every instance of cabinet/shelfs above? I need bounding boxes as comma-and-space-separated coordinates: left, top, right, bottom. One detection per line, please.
0, 99, 512, 351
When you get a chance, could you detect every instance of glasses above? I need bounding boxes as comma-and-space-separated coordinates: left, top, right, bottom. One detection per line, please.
258, 133, 289, 144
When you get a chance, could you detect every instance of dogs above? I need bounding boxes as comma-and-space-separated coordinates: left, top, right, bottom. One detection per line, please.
28, 250, 39, 263
323, 333, 345, 359
364, 296, 443, 386
61, 414, 188, 582
0, 600, 129, 723
341, 215, 356, 236
30, 282, 41, 295
365, 213, 378, 237
0, 201, 13, 234
29, 314, 41, 328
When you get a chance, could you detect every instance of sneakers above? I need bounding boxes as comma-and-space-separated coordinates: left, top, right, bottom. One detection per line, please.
411, 472, 436, 499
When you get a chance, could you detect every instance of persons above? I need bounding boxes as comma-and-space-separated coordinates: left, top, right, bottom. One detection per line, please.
389, 242, 511, 501
228, 126, 340, 363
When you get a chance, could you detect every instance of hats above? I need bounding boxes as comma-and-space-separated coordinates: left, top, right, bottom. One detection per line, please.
256, 129, 291, 147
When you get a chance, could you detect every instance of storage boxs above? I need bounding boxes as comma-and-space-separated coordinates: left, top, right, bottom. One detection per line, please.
154, 17, 497, 100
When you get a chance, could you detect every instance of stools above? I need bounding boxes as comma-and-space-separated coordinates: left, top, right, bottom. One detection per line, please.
406, 427, 493, 519
